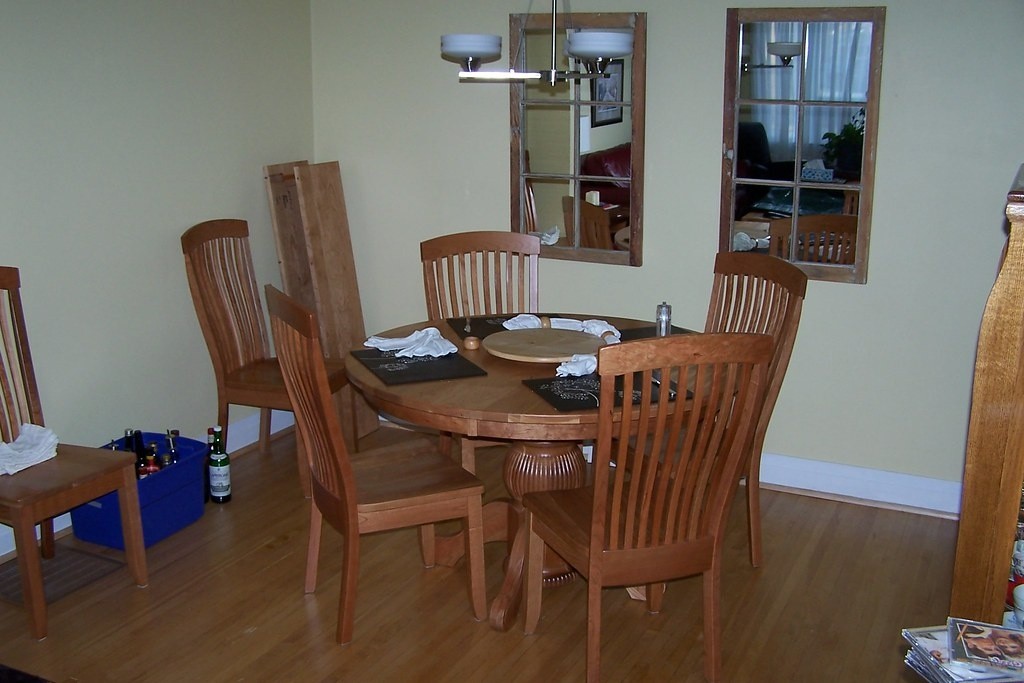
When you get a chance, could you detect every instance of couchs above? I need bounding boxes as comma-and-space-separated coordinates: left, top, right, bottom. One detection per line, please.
577, 138, 633, 206
735, 121, 772, 200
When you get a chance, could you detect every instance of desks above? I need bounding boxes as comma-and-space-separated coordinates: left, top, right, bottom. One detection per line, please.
345, 304, 745, 631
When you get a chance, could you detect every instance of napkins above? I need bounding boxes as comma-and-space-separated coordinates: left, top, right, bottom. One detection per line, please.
364, 325, 457, 358
500, 311, 621, 377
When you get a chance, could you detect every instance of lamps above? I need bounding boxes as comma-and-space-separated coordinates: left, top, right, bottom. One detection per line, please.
740, 42, 802, 70
438, 0, 634, 80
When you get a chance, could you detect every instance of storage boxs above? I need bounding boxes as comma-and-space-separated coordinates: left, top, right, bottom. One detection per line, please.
68, 431, 210, 550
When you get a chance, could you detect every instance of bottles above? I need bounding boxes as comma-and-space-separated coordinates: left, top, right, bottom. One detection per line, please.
112, 424, 179, 481
209, 425, 232, 503
657, 302, 672, 337
205, 429, 215, 453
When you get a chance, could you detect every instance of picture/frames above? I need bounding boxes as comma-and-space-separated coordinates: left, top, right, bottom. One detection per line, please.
590, 58, 624, 128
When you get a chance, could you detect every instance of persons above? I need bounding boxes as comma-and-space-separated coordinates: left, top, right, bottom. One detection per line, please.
960, 624, 1024, 659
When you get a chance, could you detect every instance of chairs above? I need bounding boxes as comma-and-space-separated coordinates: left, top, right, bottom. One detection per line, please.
670, 252, 809, 563
524, 334, 776, 681
0, 266, 149, 641
419, 231, 541, 474
180, 219, 358, 498
555, 178, 864, 267
266, 284, 490, 641
